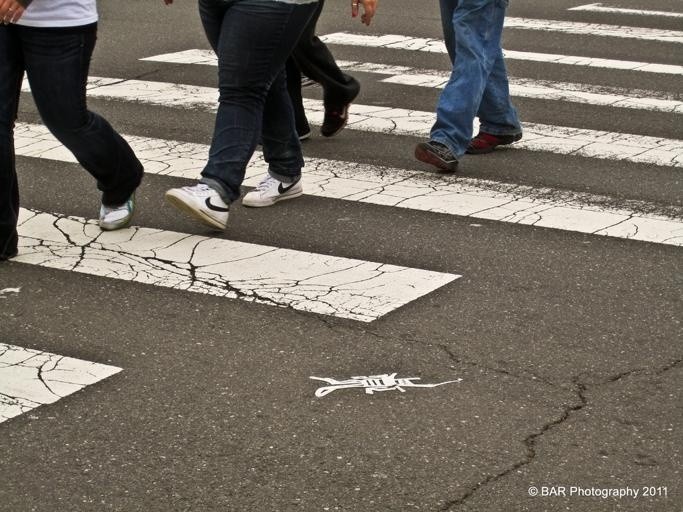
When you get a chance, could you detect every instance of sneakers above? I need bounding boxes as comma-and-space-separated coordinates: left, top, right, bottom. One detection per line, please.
98, 192, 136, 228
415, 141, 457, 174
321, 96, 349, 137
258, 117, 311, 143
465, 130, 522, 156
165, 184, 230, 230
241, 176, 304, 208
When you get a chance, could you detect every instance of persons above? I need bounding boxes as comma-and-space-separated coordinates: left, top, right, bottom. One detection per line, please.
414, 0, 523, 172
160, 0, 306, 231
289, 0, 380, 142
0, 0, 145, 264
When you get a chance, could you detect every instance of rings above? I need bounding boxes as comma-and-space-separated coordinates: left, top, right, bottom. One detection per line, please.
352, 3, 358, 6
